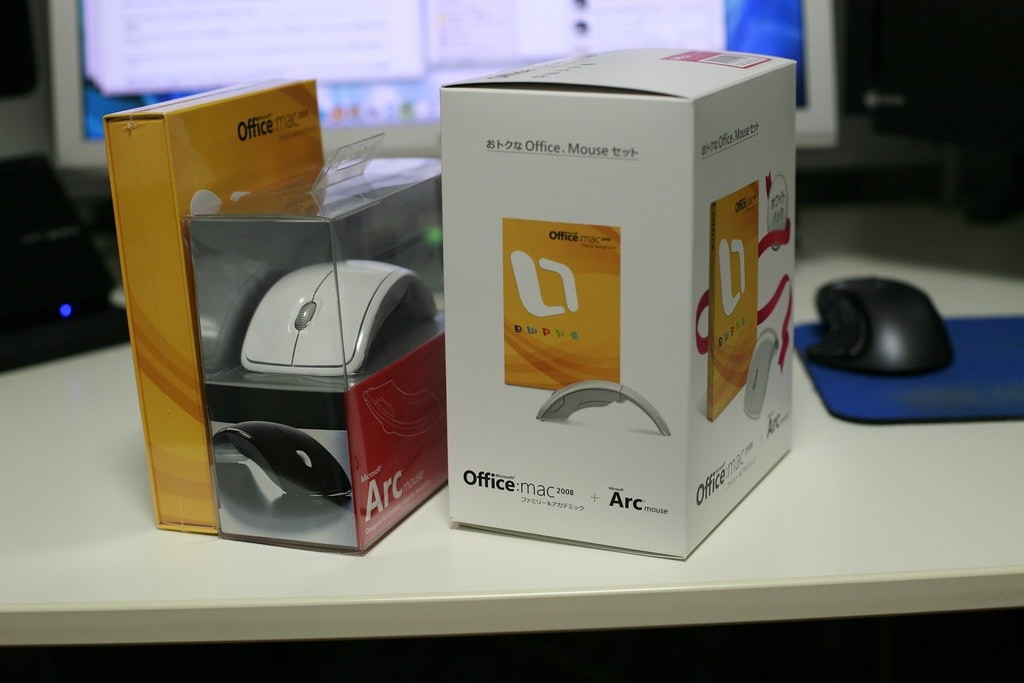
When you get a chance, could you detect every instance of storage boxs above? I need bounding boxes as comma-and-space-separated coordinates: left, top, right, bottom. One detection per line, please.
184, 133, 449, 558
439, 49, 795, 561
102, 76, 325, 536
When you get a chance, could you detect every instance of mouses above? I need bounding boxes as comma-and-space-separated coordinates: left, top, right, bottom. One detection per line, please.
239, 259, 439, 381
808, 276, 952, 375
744, 329, 779, 419
212, 421, 352, 531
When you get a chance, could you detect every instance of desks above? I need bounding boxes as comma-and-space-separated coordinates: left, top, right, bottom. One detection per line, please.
0, 205, 1023, 683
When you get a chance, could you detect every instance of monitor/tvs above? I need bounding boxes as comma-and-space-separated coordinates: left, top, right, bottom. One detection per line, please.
48, 0, 835, 170
